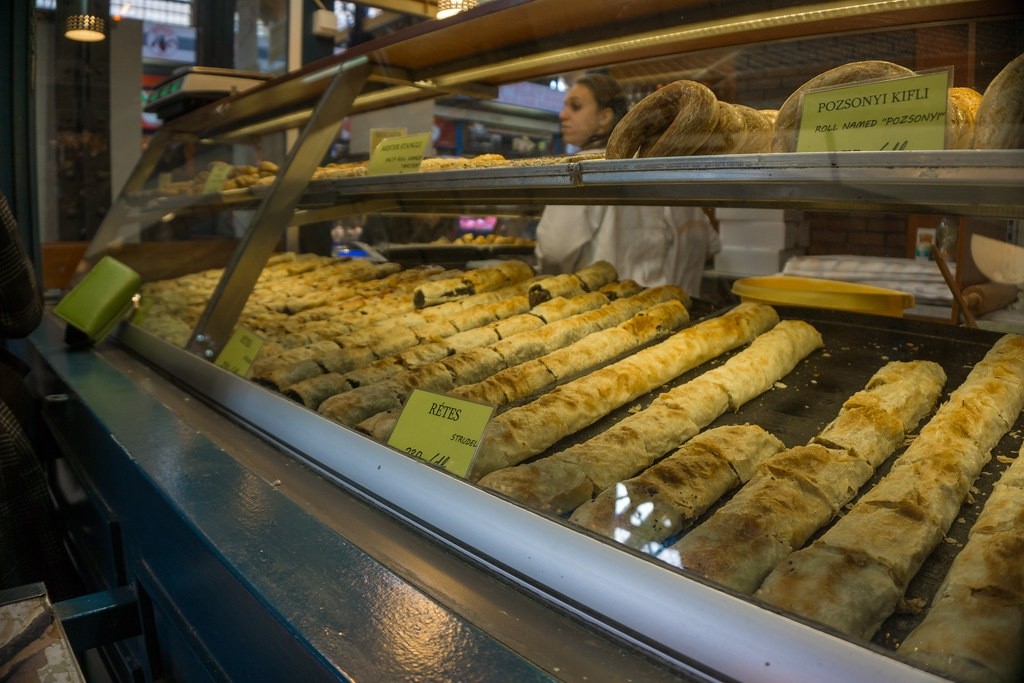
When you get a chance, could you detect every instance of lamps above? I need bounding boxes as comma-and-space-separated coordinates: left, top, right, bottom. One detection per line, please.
62, 0, 107, 42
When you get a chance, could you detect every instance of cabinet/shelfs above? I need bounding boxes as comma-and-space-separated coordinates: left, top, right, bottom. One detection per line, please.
66, 0, 1024, 683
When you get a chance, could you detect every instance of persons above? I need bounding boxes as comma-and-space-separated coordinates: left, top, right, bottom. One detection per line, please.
0, 192, 84, 597
535, 73, 721, 300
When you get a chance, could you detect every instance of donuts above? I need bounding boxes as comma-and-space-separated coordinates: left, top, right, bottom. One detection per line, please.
606, 60, 1024, 193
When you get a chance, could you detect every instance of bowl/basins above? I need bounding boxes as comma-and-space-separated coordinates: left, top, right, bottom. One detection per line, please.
732, 275, 919, 318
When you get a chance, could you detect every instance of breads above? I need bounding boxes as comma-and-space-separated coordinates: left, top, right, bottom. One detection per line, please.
146, 153, 610, 197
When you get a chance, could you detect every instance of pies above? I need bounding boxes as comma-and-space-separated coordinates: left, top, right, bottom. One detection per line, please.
123, 250, 1024, 683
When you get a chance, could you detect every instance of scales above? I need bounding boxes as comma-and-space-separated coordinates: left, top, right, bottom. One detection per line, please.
144, 66, 276, 121
